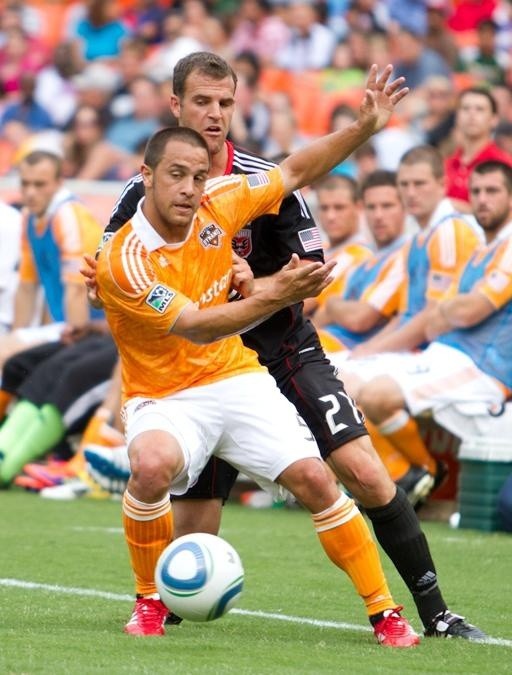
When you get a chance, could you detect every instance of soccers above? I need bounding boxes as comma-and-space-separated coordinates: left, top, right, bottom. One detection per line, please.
155, 533, 246, 622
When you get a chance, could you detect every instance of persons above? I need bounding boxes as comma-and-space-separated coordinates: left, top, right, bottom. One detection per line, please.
14, 356, 136, 500
315, 170, 417, 354
0, 312, 119, 483
323, 144, 485, 370
352, 18, 512, 142
215, 50, 379, 196
336, 160, 512, 514
96, 0, 510, 64
2, 0, 127, 148
64, 65, 178, 182
302, 175, 369, 322
0, 151, 104, 380
96, 64, 421, 649
441, 86, 512, 215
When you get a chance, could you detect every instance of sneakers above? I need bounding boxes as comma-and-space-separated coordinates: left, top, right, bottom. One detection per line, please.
164, 611, 183, 626
373, 606, 421, 649
81, 444, 134, 481
422, 609, 486, 640
410, 460, 450, 515
393, 462, 436, 509
38, 480, 88, 501
122, 592, 170, 636
23, 461, 74, 486
15, 472, 54, 491
85, 462, 129, 495
